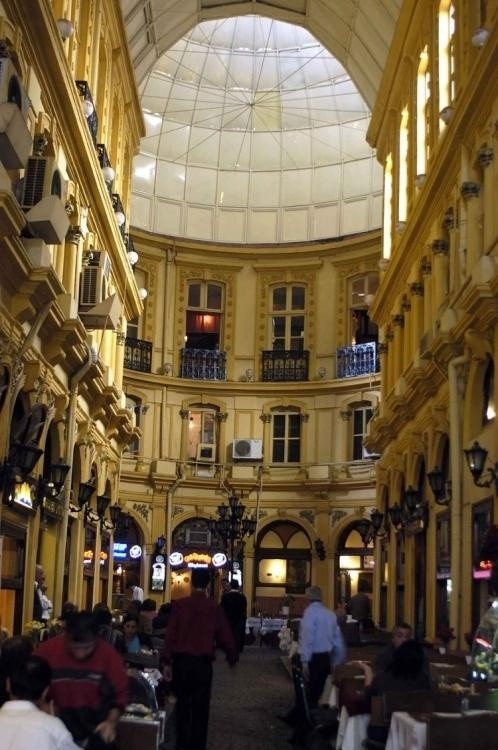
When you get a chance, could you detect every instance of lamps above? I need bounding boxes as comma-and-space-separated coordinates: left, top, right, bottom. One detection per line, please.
152, 532, 167, 556
313, 537, 326, 561
0, 432, 135, 538
352, 436, 497, 551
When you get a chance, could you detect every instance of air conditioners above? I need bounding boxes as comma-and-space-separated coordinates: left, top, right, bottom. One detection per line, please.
232, 439, 263, 460
0, 54, 117, 314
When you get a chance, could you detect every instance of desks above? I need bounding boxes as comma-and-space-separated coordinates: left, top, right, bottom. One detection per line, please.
96, 634, 196, 750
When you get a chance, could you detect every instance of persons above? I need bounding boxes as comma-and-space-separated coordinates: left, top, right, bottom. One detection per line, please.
0, 598, 169, 750
278, 579, 430, 750
129, 582, 143, 603
34, 563, 54, 622
158, 567, 246, 750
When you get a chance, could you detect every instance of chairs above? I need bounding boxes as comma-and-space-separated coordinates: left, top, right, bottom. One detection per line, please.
245, 606, 498, 750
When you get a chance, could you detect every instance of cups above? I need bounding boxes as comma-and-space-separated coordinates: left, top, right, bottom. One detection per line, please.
246, 617, 284, 630
